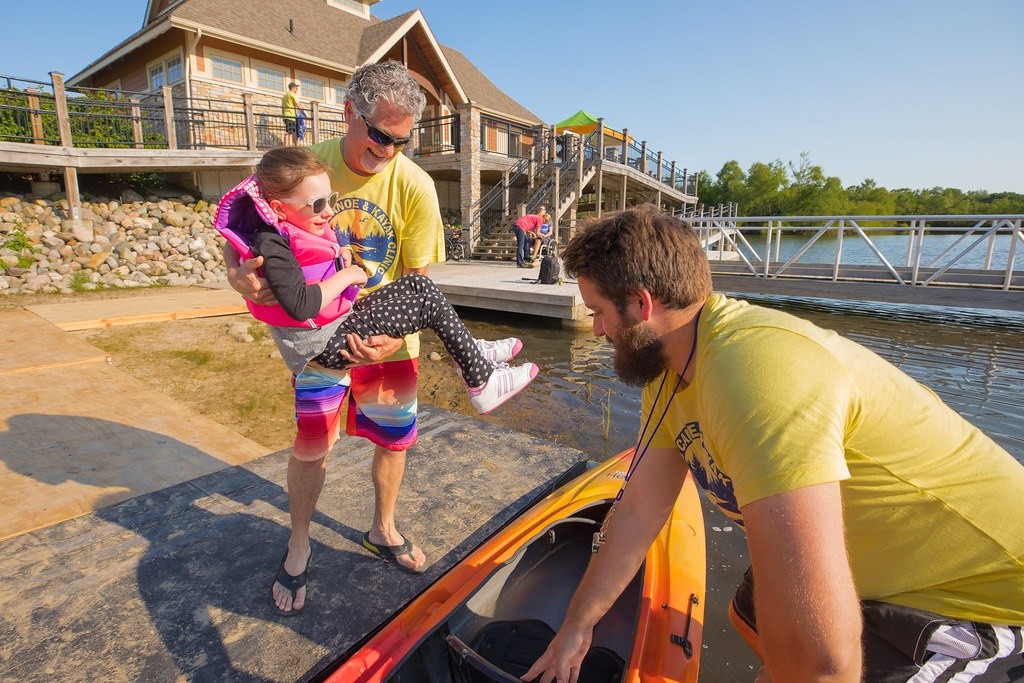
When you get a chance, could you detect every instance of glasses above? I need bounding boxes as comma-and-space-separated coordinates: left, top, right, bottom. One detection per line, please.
278, 191, 338, 215
353, 99, 413, 148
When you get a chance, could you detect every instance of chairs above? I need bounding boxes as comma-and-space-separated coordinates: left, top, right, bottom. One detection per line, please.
444, 618, 625, 683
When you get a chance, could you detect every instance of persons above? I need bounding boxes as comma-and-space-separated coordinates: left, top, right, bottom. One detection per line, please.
521, 204, 1024, 683
512, 213, 552, 268
530, 207, 553, 262
212, 146, 539, 417
224, 60, 446, 616
281, 83, 308, 148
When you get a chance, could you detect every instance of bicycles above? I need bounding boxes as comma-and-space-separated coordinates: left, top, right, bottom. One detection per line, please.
443, 228, 470, 261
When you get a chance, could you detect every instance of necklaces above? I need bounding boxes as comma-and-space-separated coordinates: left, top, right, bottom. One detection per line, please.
590, 339, 697, 555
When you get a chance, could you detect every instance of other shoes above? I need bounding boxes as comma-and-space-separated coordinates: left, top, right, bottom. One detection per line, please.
517, 263, 534, 268
525, 258, 535, 262
529, 255, 535, 262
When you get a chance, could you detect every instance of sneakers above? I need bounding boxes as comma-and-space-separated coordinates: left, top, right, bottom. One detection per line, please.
469, 360, 539, 417
454, 337, 523, 385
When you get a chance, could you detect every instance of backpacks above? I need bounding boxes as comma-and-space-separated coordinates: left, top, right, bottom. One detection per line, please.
537, 254, 560, 285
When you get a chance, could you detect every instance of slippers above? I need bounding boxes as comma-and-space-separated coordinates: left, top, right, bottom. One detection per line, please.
363, 529, 427, 577
269, 546, 312, 617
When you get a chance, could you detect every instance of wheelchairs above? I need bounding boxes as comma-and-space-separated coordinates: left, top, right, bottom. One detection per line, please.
528, 232, 558, 265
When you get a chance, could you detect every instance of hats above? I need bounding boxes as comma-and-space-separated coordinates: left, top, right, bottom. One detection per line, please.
288, 83, 300, 90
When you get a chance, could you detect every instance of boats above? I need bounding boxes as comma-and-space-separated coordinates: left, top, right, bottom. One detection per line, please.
305, 444, 708, 683
